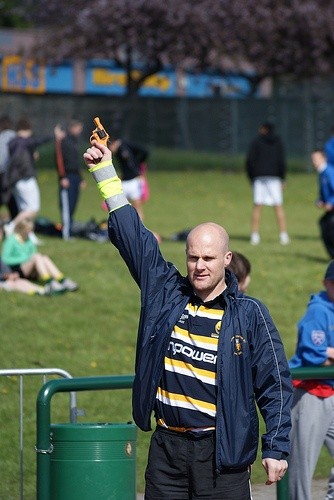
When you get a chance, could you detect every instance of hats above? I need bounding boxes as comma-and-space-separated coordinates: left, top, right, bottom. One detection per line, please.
324, 261, 334, 282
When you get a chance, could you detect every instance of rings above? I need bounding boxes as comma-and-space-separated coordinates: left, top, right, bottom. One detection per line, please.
278, 475, 285, 478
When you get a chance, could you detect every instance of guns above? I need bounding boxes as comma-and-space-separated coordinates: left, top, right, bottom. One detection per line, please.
90, 117, 111, 164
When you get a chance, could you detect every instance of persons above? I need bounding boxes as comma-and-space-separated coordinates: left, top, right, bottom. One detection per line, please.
53, 116, 83, 243
5, 117, 55, 244
243, 122, 289, 245
33, 215, 110, 244
312, 134, 334, 260
0, 178, 48, 296
286, 258, 334, 500
82, 140, 293, 500
222, 250, 251, 292
115, 130, 152, 222
0, 2, 334, 82
0, 115, 24, 222
1, 218, 78, 293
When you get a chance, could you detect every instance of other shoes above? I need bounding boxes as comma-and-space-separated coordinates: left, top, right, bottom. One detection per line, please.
249, 237, 261, 246
50, 277, 67, 294
61, 276, 79, 292
280, 238, 292, 245
34, 283, 51, 299
3, 224, 14, 240
